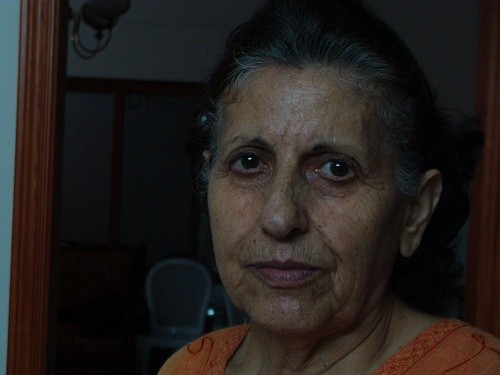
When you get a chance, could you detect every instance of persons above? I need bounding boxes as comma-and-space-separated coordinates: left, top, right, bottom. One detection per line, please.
157, 0, 500, 375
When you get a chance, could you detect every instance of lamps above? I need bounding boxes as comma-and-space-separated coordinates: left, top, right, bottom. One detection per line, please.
68, 0, 133, 61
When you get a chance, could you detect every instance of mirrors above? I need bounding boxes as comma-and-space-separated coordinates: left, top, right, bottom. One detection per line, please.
7, 0, 500, 375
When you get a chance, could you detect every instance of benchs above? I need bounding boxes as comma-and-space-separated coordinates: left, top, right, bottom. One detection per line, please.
56, 240, 147, 375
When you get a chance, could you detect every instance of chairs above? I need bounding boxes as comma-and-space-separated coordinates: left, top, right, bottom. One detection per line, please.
138, 258, 211, 375
